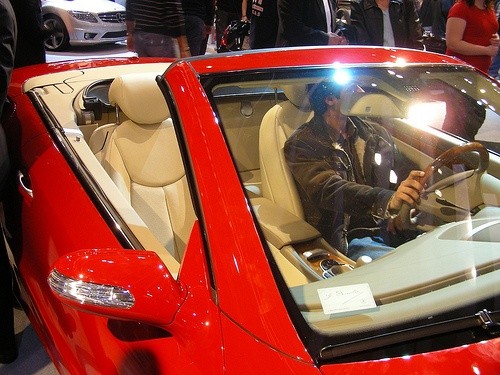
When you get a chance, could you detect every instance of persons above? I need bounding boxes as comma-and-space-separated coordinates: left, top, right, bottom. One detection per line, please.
276, 0, 346, 47
183, 0, 214, 55
442, 0, 500, 141
284, 81, 428, 260
349, 0, 424, 50
0, 0, 46, 108
125, 0, 191, 59
215, 0, 252, 52
411, 0, 454, 53
249, 0, 279, 48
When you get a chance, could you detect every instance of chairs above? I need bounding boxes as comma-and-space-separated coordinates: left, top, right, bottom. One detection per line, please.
90, 71, 200, 277
259, 80, 336, 219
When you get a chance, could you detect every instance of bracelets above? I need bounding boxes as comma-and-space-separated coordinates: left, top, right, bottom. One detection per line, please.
182, 47, 190, 51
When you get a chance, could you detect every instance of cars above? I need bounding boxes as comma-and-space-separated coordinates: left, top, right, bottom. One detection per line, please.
40, 0, 127, 52
0, 44, 500, 374
